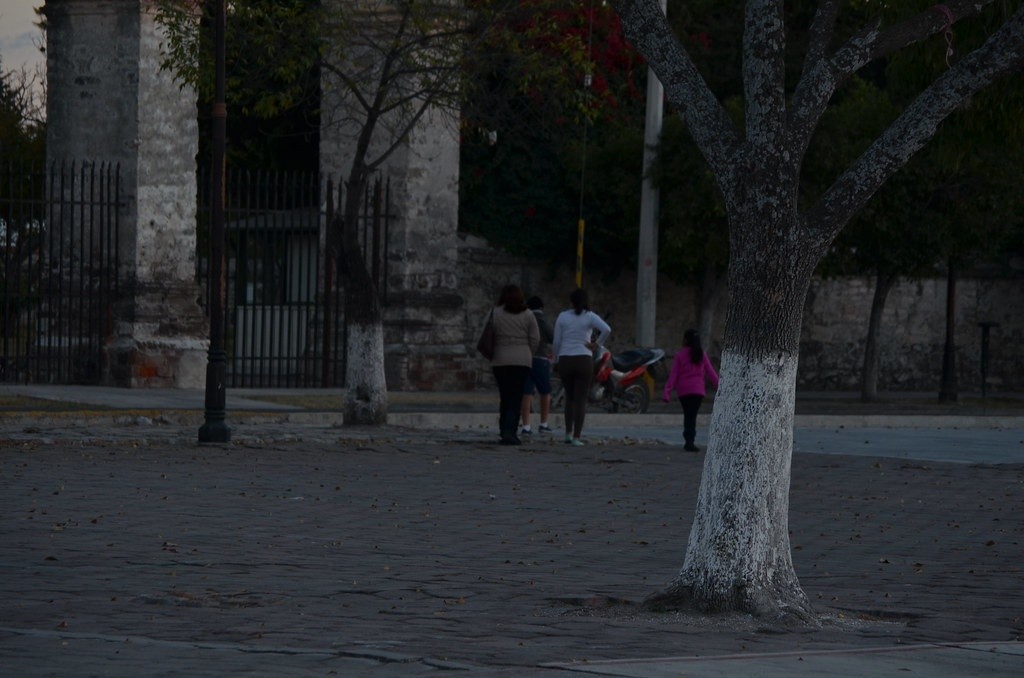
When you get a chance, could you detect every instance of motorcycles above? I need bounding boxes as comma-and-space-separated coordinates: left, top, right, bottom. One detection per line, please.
551, 337, 667, 415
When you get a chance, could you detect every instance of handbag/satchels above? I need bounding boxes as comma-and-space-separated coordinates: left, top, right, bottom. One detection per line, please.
477, 307, 494, 360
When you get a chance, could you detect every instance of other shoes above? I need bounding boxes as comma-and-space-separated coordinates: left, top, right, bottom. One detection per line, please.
571, 440, 586, 447
683, 442, 701, 452
521, 427, 533, 436
498, 429, 522, 446
564, 434, 572, 443
538, 426, 552, 433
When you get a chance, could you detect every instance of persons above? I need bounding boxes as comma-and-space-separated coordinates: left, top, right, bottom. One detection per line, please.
553, 288, 611, 446
481, 283, 540, 446
521, 297, 555, 435
663, 329, 719, 451
